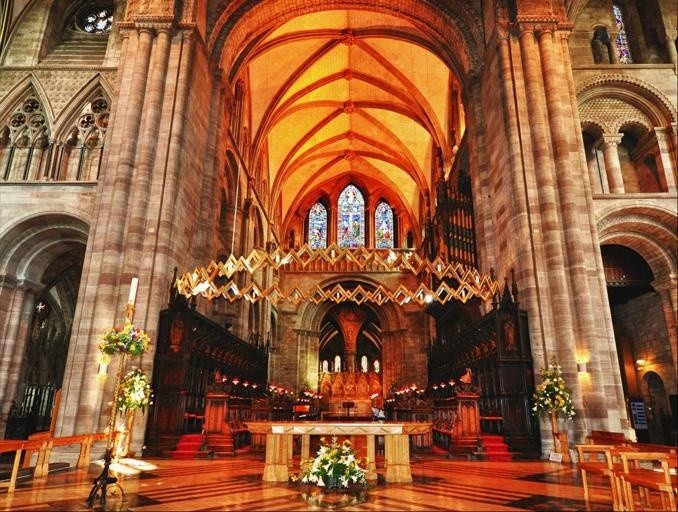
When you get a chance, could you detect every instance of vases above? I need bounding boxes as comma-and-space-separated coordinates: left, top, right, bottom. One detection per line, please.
553, 431, 572, 464
311, 486, 363, 504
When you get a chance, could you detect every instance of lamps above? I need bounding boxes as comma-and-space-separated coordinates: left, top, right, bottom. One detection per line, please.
386, 378, 455, 402
634, 359, 647, 372
221, 375, 323, 404
124, 277, 139, 324
577, 363, 587, 372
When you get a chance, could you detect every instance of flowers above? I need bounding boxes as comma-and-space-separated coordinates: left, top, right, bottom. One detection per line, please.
285, 431, 369, 487
529, 355, 576, 423
86, 323, 152, 416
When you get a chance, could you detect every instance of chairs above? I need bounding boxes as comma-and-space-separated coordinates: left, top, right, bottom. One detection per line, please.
571, 429, 678, 512
